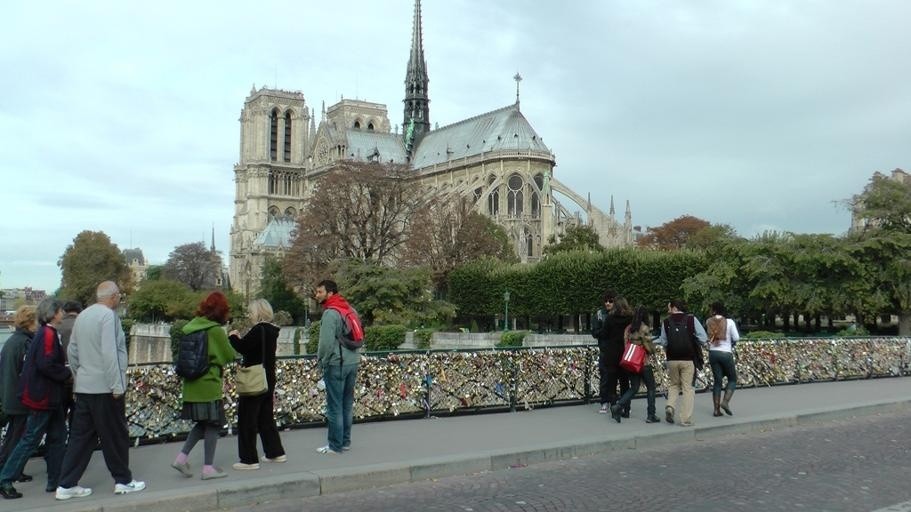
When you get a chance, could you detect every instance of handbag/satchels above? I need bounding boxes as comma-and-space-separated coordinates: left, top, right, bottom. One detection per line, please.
619, 341, 647, 374
235, 363, 268, 397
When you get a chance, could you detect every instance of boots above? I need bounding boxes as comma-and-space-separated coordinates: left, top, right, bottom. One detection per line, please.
720, 388, 733, 415
713, 393, 723, 416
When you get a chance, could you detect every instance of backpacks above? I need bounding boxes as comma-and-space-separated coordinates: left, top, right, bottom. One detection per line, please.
667, 313, 696, 358
176, 330, 222, 380
321, 304, 364, 365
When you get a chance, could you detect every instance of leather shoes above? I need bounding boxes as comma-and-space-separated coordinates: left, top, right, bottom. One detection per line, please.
16, 474, 31, 480
1, 486, 22, 499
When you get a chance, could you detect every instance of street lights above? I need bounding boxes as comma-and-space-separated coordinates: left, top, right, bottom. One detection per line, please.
502, 290, 511, 330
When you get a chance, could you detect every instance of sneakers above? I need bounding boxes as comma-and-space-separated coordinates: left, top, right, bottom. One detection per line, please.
114, 479, 145, 495
201, 470, 227, 480
599, 402, 695, 427
232, 462, 260, 470
316, 444, 350, 455
171, 460, 192, 478
55, 486, 92, 500
261, 454, 288, 463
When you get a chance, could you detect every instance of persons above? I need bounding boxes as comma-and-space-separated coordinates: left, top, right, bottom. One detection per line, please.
230, 298, 287, 470
60, 301, 84, 364
316, 280, 364, 455
170, 292, 241, 480
0, 299, 69, 498
705, 302, 740, 417
590, 290, 619, 413
612, 304, 661, 423
54, 281, 146, 500
602, 295, 633, 418
661, 299, 707, 426
0, 306, 39, 482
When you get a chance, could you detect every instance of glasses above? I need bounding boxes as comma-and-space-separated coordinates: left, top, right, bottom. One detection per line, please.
604, 299, 613, 303
